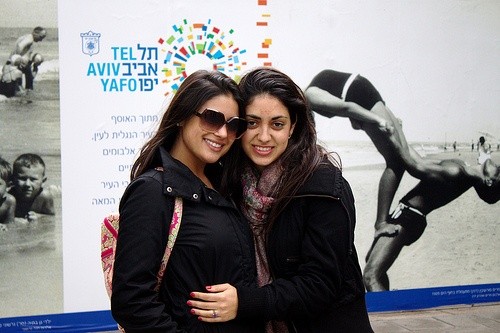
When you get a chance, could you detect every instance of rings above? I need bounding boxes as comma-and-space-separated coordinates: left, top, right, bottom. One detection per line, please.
213, 310, 216, 318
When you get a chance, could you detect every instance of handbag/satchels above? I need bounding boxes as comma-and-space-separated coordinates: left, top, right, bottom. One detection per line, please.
100, 167, 183, 333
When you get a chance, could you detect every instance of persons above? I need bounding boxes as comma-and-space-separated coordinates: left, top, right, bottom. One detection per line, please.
8, 26, 46, 90
0, 158, 16, 222
453, 136, 491, 151
105, 69, 259, 333
362, 119, 500, 291
187, 66, 374, 333
7, 153, 55, 221
0, 55, 23, 98
305, 69, 408, 234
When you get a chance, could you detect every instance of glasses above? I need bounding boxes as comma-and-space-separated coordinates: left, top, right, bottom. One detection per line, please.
194, 108, 248, 139
